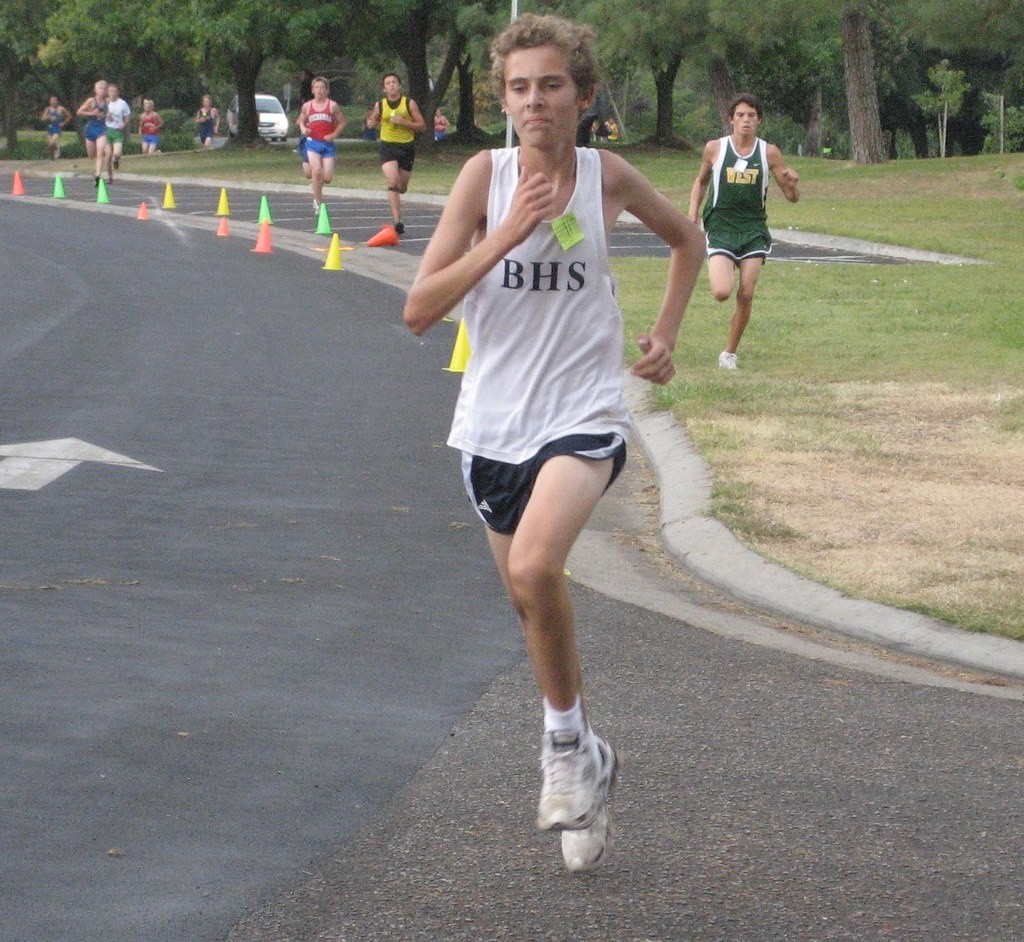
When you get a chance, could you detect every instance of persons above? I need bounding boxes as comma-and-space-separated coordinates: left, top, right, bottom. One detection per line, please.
41, 96, 72, 165
366, 73, 426, 235
403, 13, 708, 869
196, 95, 220, 150
434, 108, 449, 141
686, 95, 798, 375
588, 119, 619, 143
77, 81, 113, 188
295, 78, 346, 215
137, 99, 164, 155
104, 85, 131, 183
366, 107, 378, 139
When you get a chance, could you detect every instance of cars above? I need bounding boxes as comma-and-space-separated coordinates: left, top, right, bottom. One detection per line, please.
226, 93, 288, 143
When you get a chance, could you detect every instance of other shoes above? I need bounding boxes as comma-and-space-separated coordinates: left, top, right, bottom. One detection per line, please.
93, 175, 100, 188
54, 145, 61, 159
394, 220, 405, 234
107, 176, 112, 183
312, 199, 320, 216
114, 161, 118, 168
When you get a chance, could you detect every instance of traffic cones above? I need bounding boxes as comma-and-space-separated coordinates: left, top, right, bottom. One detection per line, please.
216, 217, 229, 236
315, 203, 333, 235
136, 202, 149, 219
216, 188, 232, 215
161, 183, 176, 208
96, 178, 112, 203
250, 219, 274, 253
255, 196, 274, 225
11, 172, 26, 195
441, 317, 473, 373
52, 175, 67, 198
367, 224, 399, 247
322, 233, 344, 270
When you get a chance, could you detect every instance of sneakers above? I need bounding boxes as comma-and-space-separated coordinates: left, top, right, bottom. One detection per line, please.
718, 351, 738, 370
561, 788, 611, 873
534, 727, 618, 831
